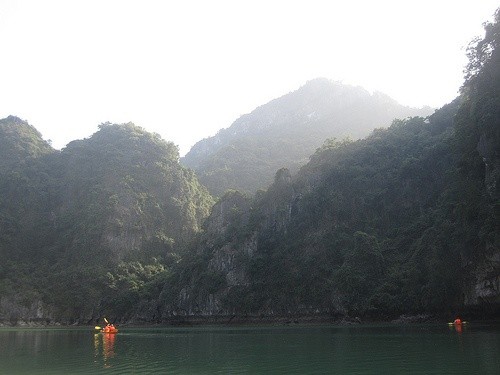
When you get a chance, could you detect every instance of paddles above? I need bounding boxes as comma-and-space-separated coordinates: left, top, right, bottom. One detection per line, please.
104, 318, 109, 324
95, 326, 103, 329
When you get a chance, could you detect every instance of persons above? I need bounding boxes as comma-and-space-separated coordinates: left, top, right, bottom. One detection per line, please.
102, 324, 115, 333
454, 317, 460, 324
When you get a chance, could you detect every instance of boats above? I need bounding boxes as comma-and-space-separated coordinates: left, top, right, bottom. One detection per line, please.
100, 329, 118, 333
448, 321, 470, 324
